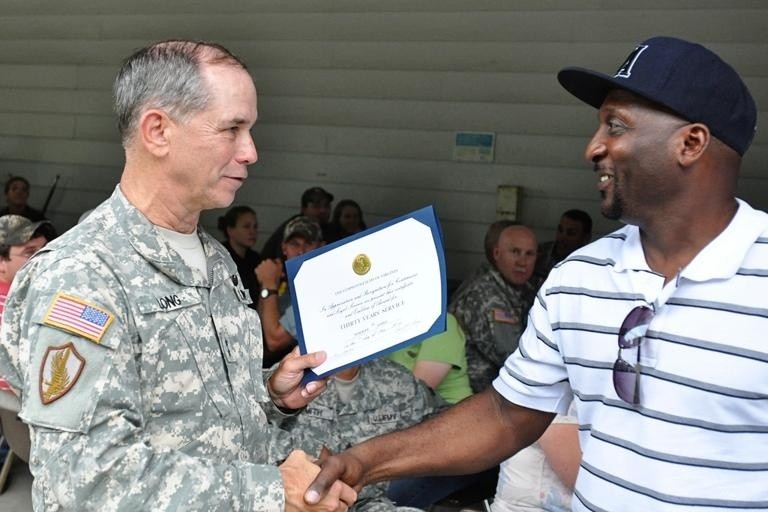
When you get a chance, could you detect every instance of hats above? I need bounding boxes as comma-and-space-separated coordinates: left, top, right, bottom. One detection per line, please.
557, 37, 756, 156
283, 187, 334, 242
0, 214, 57, 246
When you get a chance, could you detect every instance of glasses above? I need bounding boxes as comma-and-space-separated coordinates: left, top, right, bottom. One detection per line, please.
613, 306, 655, 405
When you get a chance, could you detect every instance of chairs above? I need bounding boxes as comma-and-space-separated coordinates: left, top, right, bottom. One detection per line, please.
0, 434, 15, 494
427, 474, 498, 511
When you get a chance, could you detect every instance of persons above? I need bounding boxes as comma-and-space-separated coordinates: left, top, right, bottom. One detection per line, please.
1, 34, 359, 510
218, 175, 596, 511
0, 174, 55, 390
304, 34, 767, 511
